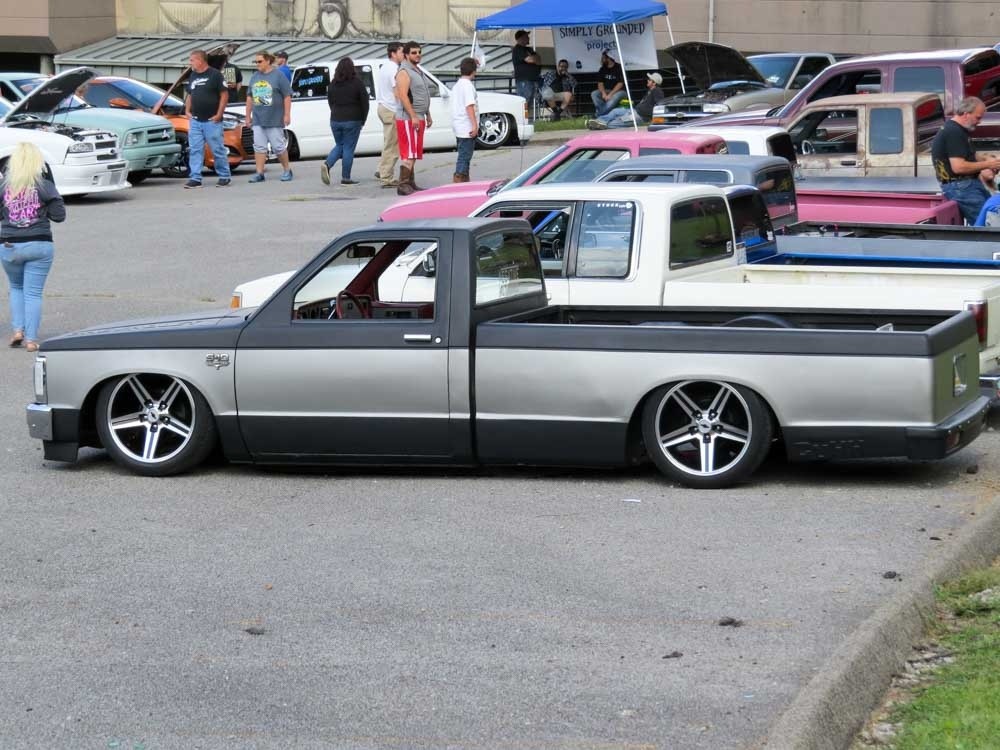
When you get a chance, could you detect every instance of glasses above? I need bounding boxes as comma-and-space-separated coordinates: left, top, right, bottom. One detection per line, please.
252, 59, 263, 63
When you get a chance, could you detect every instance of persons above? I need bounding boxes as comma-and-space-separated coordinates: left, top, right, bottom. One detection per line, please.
244, 50, 293, 183
0, 142, 67, 352
511, 29, 542, 105
450, 57, 482, 183
321, 57, 370, 187
183, 50, 243, 189
374, 40, 433, 196
585, 48, 665, 131
541, 59, 578, 121
931, 96, 1000, 228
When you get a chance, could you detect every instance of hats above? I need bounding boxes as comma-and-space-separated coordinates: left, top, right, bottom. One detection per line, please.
515, 30, 530, 40
646, 72, 663, 86
273, 50, 288, 60
600, 48, 618, 64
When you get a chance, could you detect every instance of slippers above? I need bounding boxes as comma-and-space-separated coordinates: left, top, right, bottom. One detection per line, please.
26, 343, 40, 351
7, 335, 21, 347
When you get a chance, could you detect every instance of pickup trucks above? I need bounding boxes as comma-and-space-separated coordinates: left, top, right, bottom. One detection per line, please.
25, 216, 992, 488
215, 57, 538, 162
231, 46, 1000, 413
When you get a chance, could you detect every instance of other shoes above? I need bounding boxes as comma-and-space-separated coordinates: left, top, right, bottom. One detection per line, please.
321, 164, 330, 184
552, 111, 560, 122
341, 179, 361, 185
216, 178, 231, 187
584, 119, 607, 130
379, 178, 399, 187
246, 173, 264, 183
594, 107, 601, 118
183, 178, 202, 188
280, 168, 293, 179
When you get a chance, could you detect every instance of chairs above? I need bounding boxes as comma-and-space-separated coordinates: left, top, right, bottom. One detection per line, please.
538, 73, 554, 121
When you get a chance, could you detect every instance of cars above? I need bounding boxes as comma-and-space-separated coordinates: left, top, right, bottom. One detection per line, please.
646, 40, 868, 132
0, 66, 258, 202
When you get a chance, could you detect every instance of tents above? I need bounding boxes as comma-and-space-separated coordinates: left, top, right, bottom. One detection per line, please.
470, 0, 687, 134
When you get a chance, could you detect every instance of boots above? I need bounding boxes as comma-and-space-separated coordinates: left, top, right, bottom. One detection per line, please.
397, 165, 415, 195
453, 172, 469, 182
409, 165, 428, 192
